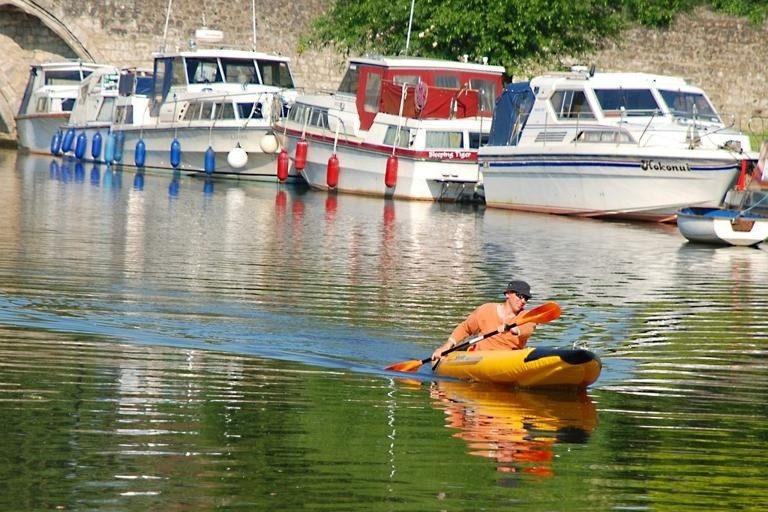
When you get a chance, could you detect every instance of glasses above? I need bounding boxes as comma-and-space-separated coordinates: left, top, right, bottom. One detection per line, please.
516, 293, 528, 302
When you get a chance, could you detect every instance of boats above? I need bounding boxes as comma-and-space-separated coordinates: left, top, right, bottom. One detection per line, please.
14, 61, 118, 156
432, 347, 602, 388
476, 64, 761, 224
272, 57, 506, 205
51, 12, 302, 177
675, 205, 768, 246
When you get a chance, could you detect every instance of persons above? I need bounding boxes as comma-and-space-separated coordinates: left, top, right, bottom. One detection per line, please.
431, 389, 535, 489
431, 279, 537, 362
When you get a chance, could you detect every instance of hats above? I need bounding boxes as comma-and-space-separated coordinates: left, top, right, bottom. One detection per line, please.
503, 280, 533, 298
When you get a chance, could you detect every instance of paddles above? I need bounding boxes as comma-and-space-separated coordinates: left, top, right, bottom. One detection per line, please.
382, 302, 563, 374
